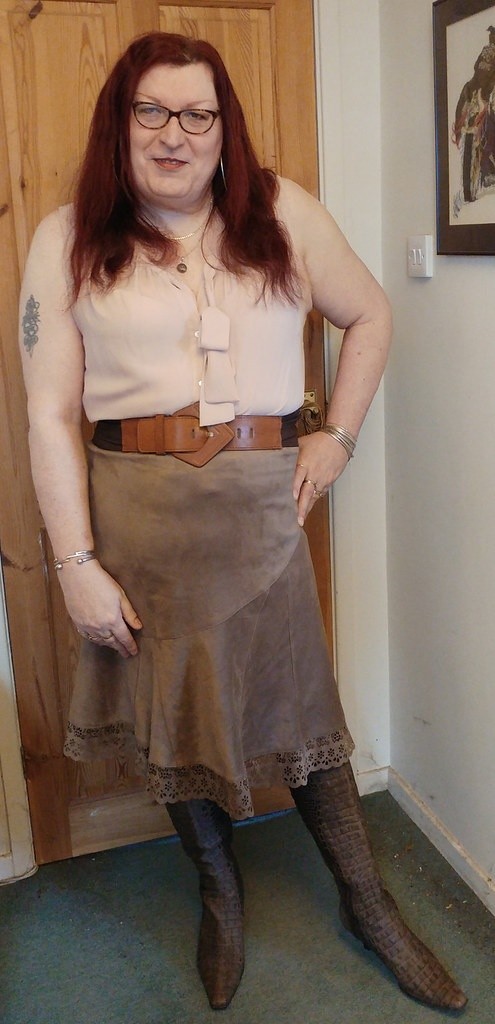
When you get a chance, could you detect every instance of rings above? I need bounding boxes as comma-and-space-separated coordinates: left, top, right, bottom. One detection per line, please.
103, 633, 114, 640
88, 635, 100, 641
319, 492, 327, 497
313, 490, 321, 495
296, 464, 310, 474
303, 479, 318, 488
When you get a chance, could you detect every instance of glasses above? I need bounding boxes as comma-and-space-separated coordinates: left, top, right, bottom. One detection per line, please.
131, 100, 220, 135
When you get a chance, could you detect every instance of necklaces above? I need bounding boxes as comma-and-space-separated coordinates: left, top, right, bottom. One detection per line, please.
141, 187, 221, 273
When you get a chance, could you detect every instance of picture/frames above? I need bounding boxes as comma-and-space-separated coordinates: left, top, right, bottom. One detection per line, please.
431, 0, 495, 256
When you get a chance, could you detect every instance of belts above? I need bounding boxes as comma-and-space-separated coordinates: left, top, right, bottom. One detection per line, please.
93, 411, 303, 468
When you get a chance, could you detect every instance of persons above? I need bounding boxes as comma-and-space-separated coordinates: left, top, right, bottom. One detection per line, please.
21, 25, 470, 1012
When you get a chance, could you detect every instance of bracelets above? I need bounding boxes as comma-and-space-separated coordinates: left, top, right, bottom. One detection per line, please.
318, 421, 358, 462
53, 548, 98, 573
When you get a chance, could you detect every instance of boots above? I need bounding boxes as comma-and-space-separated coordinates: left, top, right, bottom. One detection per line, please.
283, 761, 467, 1009
165, 798, 244, 1009
